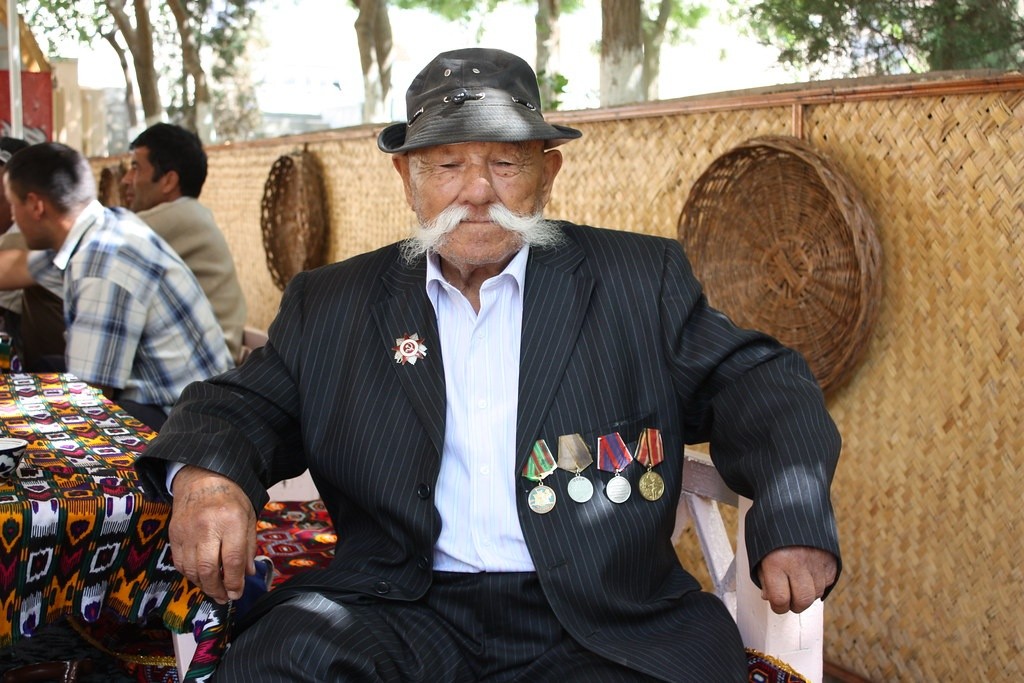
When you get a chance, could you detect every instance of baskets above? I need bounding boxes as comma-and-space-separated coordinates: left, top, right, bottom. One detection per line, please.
259, 148, 328, 292
677, 135, 885, 395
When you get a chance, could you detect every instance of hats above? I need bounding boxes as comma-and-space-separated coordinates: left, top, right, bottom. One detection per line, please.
375, 48, 583, 154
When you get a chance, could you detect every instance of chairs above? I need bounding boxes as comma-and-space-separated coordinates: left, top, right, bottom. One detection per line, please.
239, 326, 270, 365
665, 448, 825, 680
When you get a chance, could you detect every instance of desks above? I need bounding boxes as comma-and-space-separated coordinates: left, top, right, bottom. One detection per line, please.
1, 373, 175, 655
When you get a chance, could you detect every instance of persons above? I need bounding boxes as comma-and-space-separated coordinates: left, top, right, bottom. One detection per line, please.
1, 135, 36, 360
1, 139, 240, 435
132, 47, 844, 683
116, 120, 247, 369
